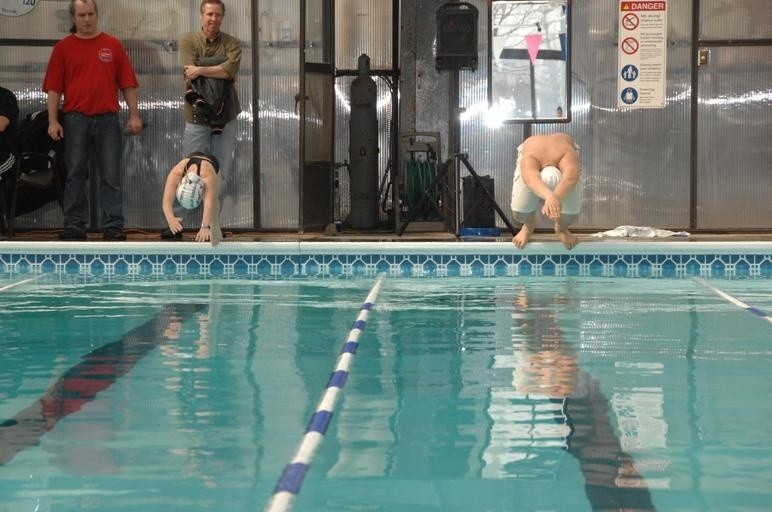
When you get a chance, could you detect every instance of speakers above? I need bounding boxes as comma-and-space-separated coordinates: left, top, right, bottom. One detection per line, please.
436, 2, 478, 68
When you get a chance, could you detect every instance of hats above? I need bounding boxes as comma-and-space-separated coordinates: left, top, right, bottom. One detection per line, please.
540, 166, 561, 190
175, 173, 204, 210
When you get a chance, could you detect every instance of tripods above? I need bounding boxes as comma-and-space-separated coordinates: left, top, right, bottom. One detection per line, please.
397, 68, 517, 238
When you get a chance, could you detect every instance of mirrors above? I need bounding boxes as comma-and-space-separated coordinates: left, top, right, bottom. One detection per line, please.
487, 2, 572, 125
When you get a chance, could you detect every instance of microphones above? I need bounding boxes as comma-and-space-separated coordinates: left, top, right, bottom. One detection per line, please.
124, 123, 147, 136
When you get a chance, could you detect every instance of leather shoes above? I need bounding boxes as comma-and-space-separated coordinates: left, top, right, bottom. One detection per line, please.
59, 227, 127, 240
160, 228, 182, 239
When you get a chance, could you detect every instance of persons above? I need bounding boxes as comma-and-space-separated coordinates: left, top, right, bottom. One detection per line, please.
0, 86, 19, 240
42, 0, 144, 242
159, 1, 244, 247
510, 134, 583, 251
